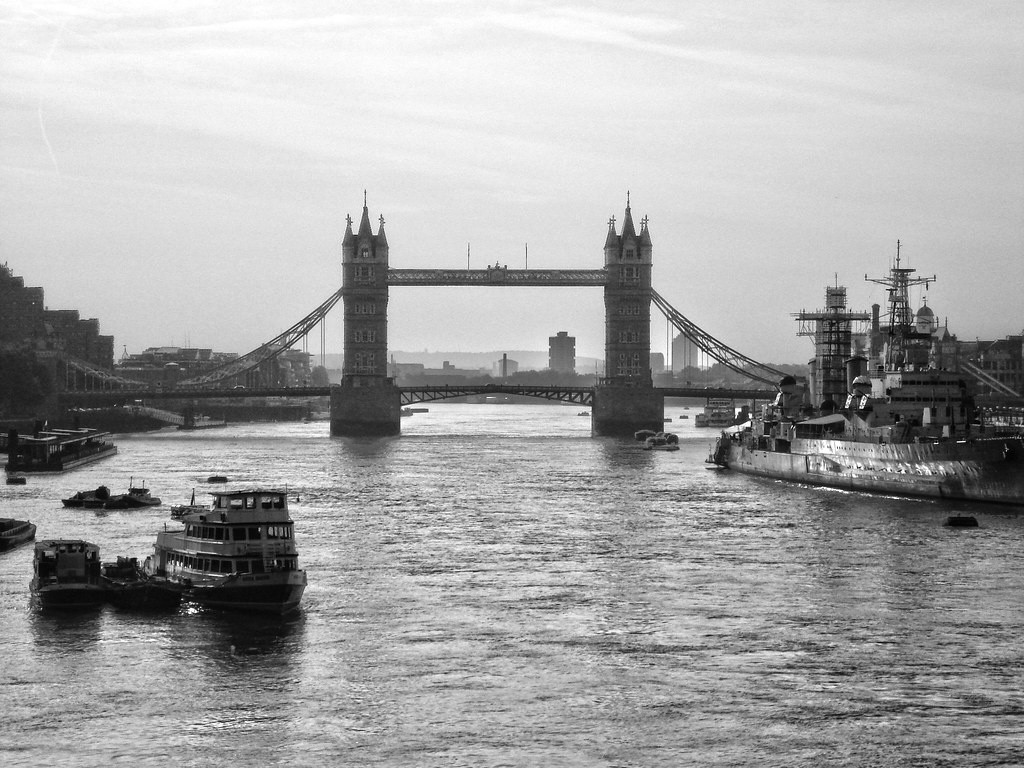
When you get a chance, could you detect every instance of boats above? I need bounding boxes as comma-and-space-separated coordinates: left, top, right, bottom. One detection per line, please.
0, 413, 314, 611
701, 239, 1024, 505
695, 396, 735, 428
